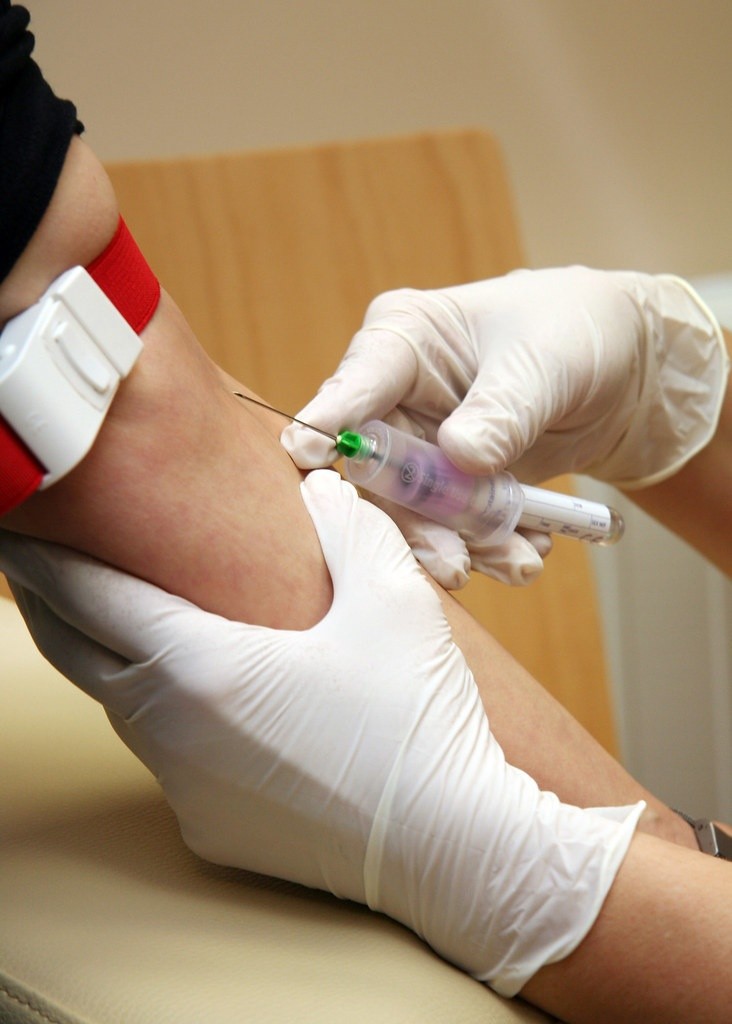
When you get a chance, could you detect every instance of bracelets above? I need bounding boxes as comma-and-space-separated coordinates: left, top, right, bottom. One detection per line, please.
671, 796, 732, 867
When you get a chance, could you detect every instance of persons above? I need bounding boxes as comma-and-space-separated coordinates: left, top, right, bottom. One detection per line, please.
3, 257, 732, 1024
2, 3, 732, 873
